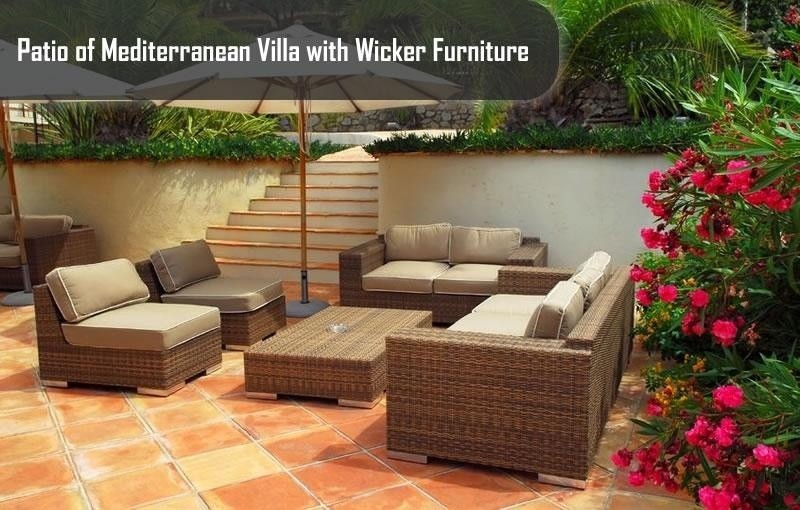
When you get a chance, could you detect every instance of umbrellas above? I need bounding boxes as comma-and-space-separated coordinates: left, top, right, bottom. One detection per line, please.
125, 19, 463, 304
0, 37, 145, 291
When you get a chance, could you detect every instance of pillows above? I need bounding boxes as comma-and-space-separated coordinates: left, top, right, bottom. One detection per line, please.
383, 221, 454, 264
569, 248, 613, 313
43, 258, 151, 325
446, 225, 524, 267
520, 278, 587, 341
148, 239, 222, 295
14, 214, 74, 244
0, 213, 16, 243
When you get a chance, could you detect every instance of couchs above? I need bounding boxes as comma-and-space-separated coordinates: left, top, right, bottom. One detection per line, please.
30, 257, 224, 399
383, 263, 637, 492
336, 222, 550, 326
133, 238, 287, 350
0, 213, 100, 292
